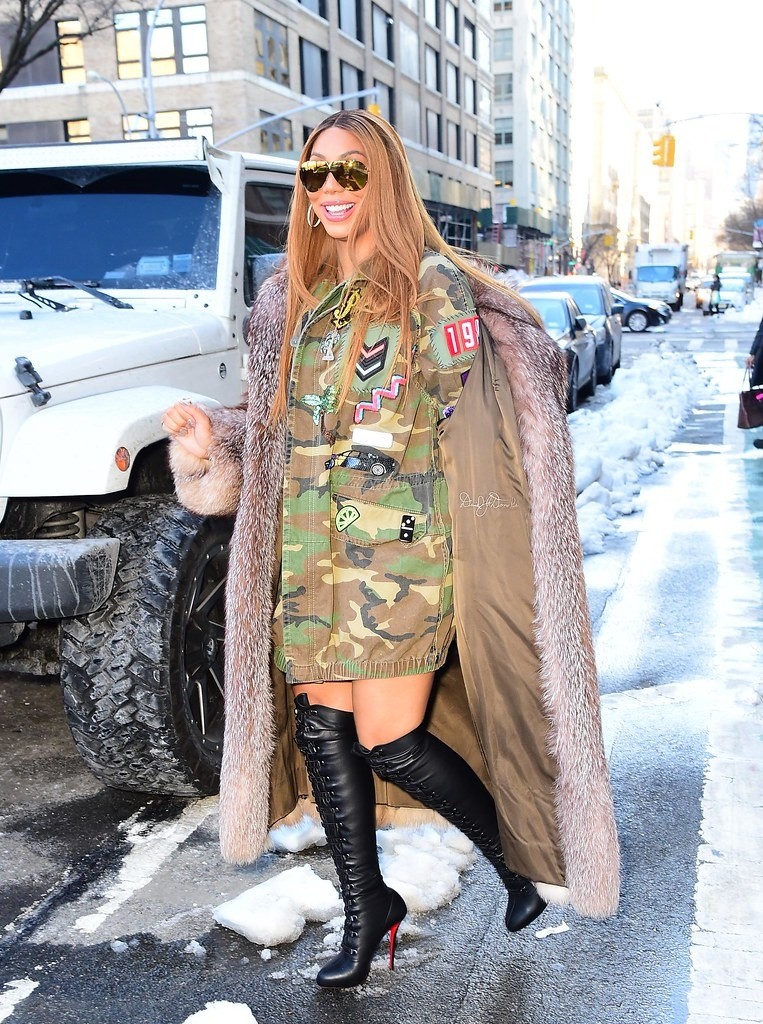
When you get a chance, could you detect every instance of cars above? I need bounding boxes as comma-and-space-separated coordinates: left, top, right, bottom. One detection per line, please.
693, 269, 754, 316
518, 292, 596, 414
521, 276, 623, 385
586, 287, 672, 332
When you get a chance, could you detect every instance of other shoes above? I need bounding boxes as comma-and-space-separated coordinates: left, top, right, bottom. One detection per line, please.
753, 439, 763, 449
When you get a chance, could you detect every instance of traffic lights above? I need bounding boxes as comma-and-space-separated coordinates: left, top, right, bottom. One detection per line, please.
653, 137, 663, 166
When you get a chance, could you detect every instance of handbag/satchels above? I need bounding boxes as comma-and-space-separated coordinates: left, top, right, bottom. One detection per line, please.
737, 365, 763, 429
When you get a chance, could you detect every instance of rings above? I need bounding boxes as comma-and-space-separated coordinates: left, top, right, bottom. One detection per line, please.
161, 422, 164, 425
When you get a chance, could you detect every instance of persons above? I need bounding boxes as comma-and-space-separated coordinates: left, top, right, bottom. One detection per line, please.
709, 275, 722, 315
158, 110, 621, 986
745, 316, 763, 449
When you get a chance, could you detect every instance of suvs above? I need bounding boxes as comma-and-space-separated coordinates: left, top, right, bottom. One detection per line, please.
0, 138, 313, 801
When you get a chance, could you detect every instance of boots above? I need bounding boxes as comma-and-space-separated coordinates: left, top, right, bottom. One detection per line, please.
353, 722, 550, 932
293, 693, 407, 988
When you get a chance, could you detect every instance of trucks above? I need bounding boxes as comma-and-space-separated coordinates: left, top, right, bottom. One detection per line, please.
628, 242, 689, 312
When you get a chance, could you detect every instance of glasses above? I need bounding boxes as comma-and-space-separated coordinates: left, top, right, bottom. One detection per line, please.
299, 159, 370, 192
